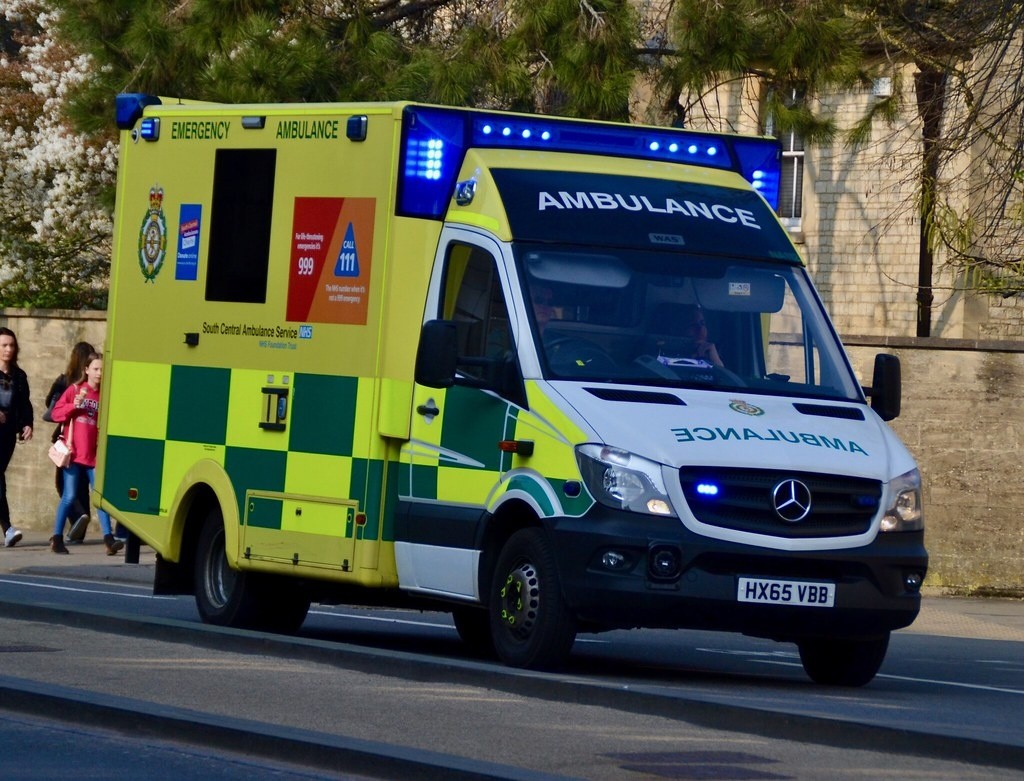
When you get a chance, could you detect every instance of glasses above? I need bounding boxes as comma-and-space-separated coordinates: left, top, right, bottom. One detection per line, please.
533, 296, 555, 306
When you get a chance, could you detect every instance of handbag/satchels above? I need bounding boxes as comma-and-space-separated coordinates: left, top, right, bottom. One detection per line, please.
48, 439, 71, 468
43, 393, 60, 422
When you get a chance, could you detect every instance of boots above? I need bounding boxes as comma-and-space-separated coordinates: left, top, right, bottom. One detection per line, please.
48, 534, 69, 554
103, 534, 123, 556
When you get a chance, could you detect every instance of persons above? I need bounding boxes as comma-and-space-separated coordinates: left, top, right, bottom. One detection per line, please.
50, 353, 123, 556
44, 341, 97, 538
464, 285, 566, 381
0, 327, 34, 548
670, 305, 726, 369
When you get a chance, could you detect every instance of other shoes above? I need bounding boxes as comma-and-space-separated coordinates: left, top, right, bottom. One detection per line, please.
63, 539, 84, 547
68, 514, 88, 539
5, 527, 22, 547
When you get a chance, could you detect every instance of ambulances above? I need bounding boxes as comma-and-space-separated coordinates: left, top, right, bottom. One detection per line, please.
87, 93, 931, 687
